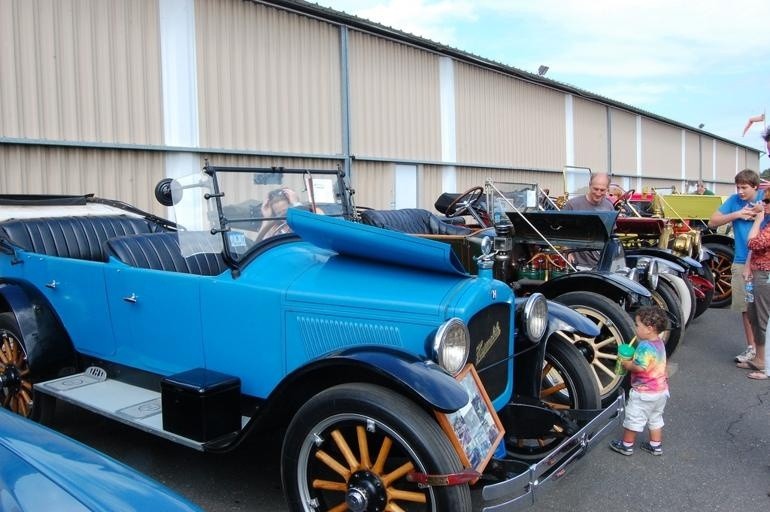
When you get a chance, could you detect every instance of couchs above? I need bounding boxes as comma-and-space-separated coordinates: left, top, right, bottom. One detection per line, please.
360, 208, 472, 237
108, 229, 254, 275
252, 202, 344, 233
0, 216, 166, 262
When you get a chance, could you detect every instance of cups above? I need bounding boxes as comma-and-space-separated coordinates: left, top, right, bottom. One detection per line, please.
616, 345, 635, 377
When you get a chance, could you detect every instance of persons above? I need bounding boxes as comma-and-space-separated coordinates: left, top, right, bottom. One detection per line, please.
688, 181, 716, 229
251, 187, 324, 244
560, 171, 614, 212
608, 306, 670, 456
707, 169, 770, 362
743, 179, 770, 379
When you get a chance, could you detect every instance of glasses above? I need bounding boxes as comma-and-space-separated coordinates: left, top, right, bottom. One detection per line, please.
762, 198, 770, 203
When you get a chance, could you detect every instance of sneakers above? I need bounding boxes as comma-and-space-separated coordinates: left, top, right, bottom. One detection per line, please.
734, 345, 770, 380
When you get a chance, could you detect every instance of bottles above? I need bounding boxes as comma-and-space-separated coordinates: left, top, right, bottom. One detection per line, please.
744, 276, 754, 302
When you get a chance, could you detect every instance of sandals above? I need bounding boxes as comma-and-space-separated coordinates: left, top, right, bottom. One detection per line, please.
609, 440, 634, 455
641, 440, 663, 455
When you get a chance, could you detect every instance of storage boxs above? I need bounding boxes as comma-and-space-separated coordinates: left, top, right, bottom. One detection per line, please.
160, 368, 242, 442
